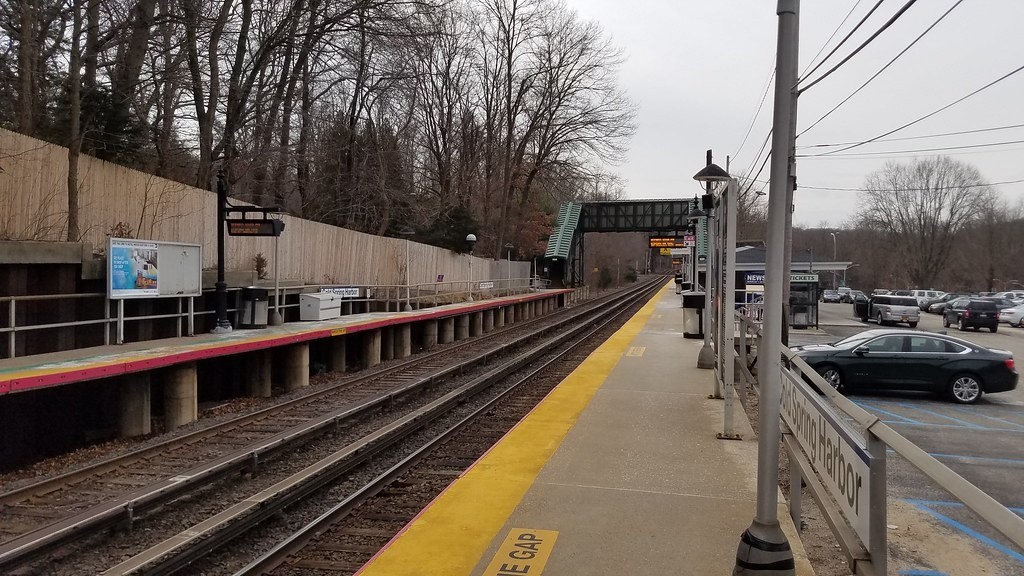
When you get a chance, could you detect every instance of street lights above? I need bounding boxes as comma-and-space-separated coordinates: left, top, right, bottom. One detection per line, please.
693, 164, 732, 370
398, 226, 416, 310
830, 232, 840, 289
466, 234, 476, 302
844, 264, 861, 288
504, 243, 514, 288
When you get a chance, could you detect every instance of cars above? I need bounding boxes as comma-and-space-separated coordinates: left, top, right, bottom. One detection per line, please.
781, 328, 1019, 405
853, 292, 921, 328
820, 284, 1024, 327
943, 299, 1000, 333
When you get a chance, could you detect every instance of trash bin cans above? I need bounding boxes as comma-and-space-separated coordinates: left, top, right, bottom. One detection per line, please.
793, 306, 808, 329
682, 291, 706, 339
681, 282, 691, 292
240, 285, 269, 329
300, 292, 341, 320
675, 278, 682, 294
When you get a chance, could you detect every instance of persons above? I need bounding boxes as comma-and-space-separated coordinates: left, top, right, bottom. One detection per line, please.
674, 270, 682, 294
